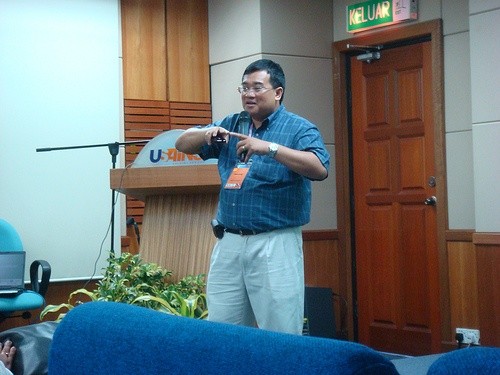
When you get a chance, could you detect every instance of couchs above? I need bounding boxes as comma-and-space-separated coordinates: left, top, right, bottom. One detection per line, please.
49, 301, 500, 375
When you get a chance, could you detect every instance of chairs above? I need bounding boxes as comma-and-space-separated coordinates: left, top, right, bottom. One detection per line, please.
0, 219, 51, 323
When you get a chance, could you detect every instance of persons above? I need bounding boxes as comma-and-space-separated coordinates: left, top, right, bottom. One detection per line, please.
0, 339, 18, 375
174, 59, 330, 336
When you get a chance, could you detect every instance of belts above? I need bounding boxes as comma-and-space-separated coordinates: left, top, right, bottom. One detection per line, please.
226, 228, 266, 236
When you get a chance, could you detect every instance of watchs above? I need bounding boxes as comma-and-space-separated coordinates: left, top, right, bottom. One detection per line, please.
268, 143, 279, 159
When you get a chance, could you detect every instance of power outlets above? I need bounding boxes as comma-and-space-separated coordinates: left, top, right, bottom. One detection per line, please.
455, 327, 481, 345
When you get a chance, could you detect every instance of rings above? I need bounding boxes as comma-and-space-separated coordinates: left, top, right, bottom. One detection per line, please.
5, 353, 9, 357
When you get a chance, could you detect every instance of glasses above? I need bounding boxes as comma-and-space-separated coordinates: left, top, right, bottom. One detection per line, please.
237, 86, 276, 95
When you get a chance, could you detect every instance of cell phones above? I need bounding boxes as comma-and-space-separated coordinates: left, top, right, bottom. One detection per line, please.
211, 134, 226, 143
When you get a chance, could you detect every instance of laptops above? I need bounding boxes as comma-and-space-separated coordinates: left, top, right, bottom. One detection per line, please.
0, 251, 26, 295
